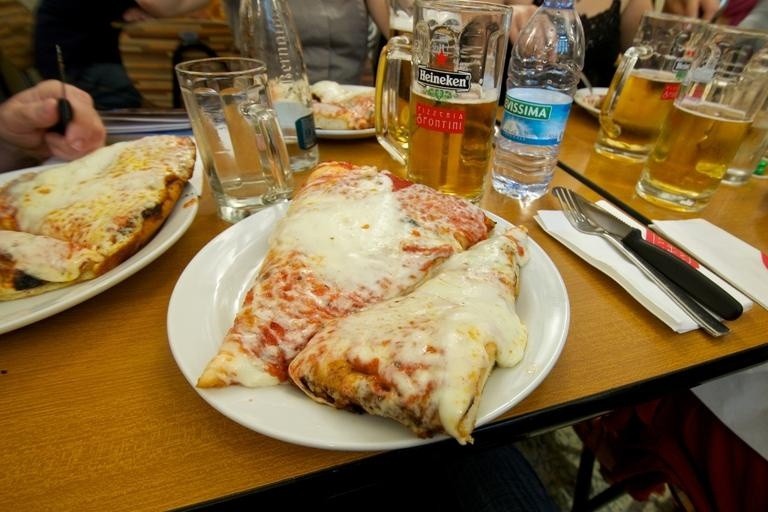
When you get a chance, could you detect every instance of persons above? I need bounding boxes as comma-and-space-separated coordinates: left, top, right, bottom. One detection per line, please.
0, 79, 107, 173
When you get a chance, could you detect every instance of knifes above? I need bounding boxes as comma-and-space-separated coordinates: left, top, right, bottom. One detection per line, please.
565, 189, 745, 322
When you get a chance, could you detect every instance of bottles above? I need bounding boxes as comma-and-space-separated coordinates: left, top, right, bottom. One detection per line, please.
231, 2, 320, 175
488, 0, 588, 202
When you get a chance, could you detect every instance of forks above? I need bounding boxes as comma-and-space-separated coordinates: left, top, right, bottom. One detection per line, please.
549, 187, 728, 339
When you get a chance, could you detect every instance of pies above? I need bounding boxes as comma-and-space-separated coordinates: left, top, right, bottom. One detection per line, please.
197, 158, 498, 398
287, 223, 528, 447
267, 79, 376, 132
0, 131, 197, 303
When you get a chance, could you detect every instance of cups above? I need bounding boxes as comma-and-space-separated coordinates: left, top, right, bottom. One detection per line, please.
174, 54, 296, 224
373, 0, 513, 205
591, 8, 768, 211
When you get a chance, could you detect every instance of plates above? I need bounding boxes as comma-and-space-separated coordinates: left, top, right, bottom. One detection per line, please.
570, 84, 615, 119
165, 171, 573, 455
309, 83, 397, 138
0, 159, 200, 336
